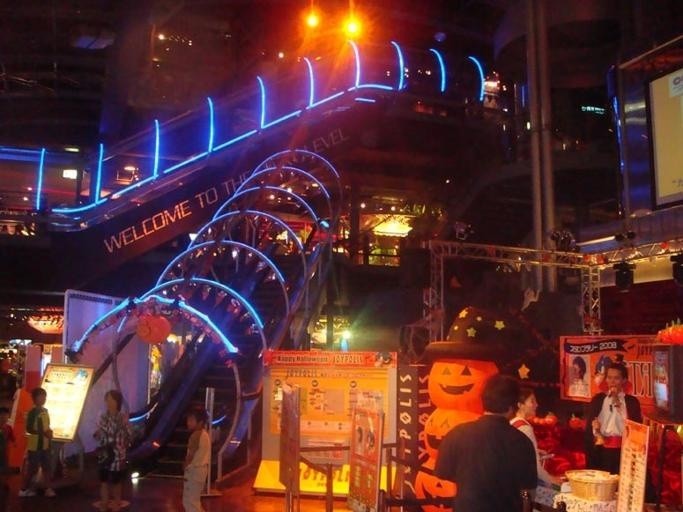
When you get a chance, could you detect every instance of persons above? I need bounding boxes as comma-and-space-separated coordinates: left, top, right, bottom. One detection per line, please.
435, 375, 538, 512
584, 362, 641, 476
180, 403, 211, 512
19, 388, 57, 497
0, 342, 26, 475
508, 385, 562, 487
90, 390, 134, 511
567, 357, 591, 399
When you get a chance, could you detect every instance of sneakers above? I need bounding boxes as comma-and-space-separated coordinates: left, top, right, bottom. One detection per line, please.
44, 488, 57, 497
18, 488, 36, 497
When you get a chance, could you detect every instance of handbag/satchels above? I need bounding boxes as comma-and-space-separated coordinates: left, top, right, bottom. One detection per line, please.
95, 440, 116, 465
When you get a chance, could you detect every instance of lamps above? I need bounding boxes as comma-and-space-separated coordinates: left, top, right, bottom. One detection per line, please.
612, 262, 636, 293
668, 255, 683, 284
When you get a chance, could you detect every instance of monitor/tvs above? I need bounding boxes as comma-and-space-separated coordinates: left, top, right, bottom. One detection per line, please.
651, 344, 683, 421
642, 64, 683, 211
